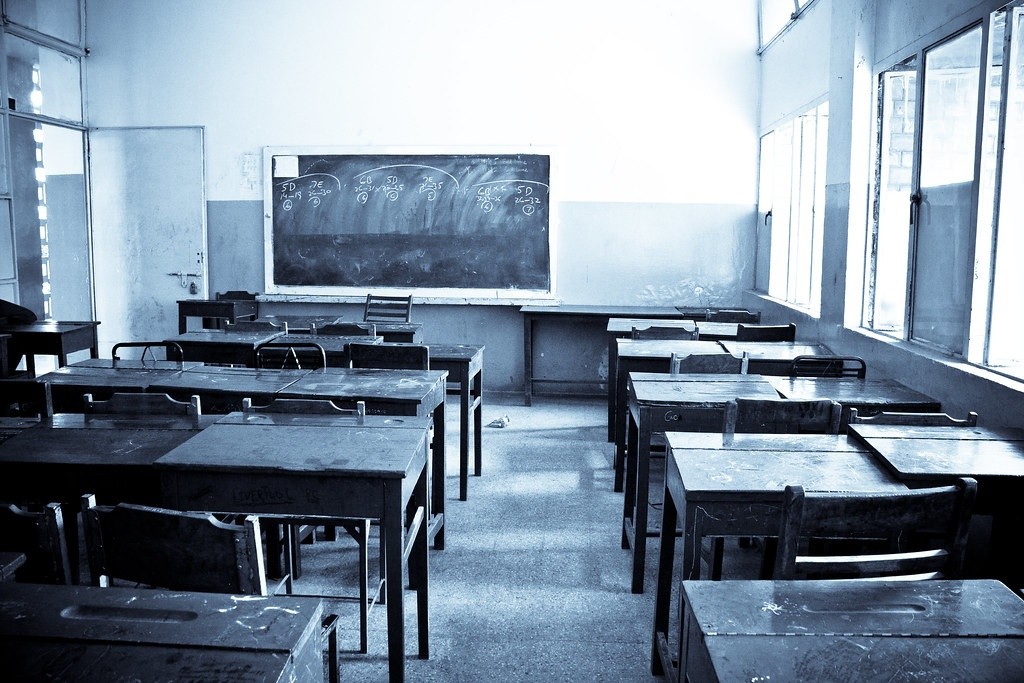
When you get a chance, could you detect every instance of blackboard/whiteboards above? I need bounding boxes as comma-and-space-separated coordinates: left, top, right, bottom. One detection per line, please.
261, 144, 561, 300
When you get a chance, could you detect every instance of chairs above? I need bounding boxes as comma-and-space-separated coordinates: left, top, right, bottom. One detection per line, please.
80, 492, 340, 683
241, 394, 391, 652
700, 397, 842, 579
342, 344, 430, 375
112, 343, 184, 363
705, 308, 761, 324
1, 502, 72, 584
218, 321, 287, 367
737, 323, 797, 341
847, 407, 979, 426
81, 390, 242, 591
790, 354, 866, 378
363, 294, 413, 322
254, 342, 327, 369
631, 325, 700, 339
0, 333, 34, 377
0, 379, 53, 421
773, 476, 978, 579
216, 292, 258, 329
670, 350, 749, 373
312, 323, 377, 336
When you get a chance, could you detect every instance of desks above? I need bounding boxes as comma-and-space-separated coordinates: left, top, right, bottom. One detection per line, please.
651, 433, 911, 673
676, 304, 750, 321
697, 320, 763, 339
0, 412, 226, 595
848, 422, 1024, 600
679, 579, 1024, 682
16, 320, 100, 376
276, 367, 450, 588
253, 314, 345, 334
719, 339, 844, 375
0, 583, 323, 682
608, 317, 695, 440
0, 549, 27, 583
614, 338, 726, 492
257, 334, 383, 366
378, 341, 485, 501
151, 364, 313, 579
620, 372, 782, 593
151, 410, 432, 683
761, 375, 940, 434
519, 305, 683, 405
33, 357, 206, 412
0, 408, 42, 446
164, 327, 286, 368
337, 320, 424, 343
177, 299, 259, 335
